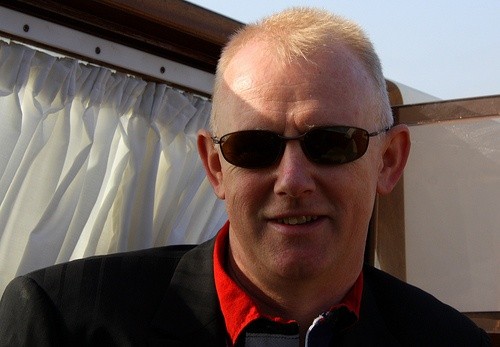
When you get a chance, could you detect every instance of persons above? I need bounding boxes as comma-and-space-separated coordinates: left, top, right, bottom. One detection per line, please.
0, 4, 500, 347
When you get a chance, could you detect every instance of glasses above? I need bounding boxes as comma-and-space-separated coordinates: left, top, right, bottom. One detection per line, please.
211, 126, 390, 169
